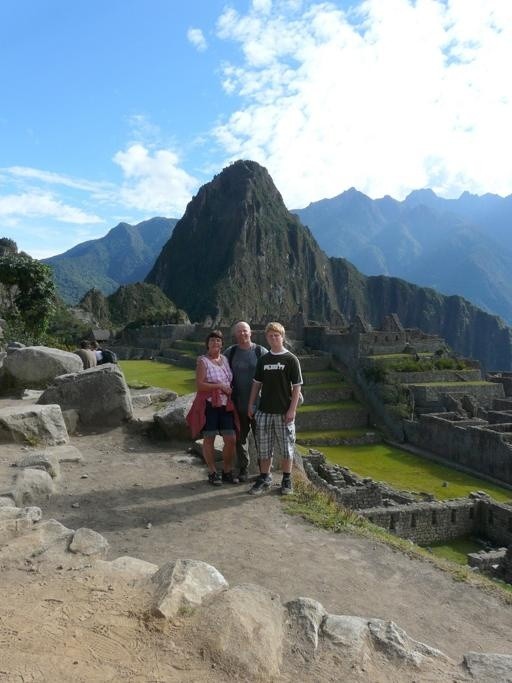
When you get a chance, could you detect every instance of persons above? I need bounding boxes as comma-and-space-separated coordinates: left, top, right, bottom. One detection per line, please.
91, 340, 105, 365
195, 329, 241, 485
75, 339, 97, 370
223, 321, 269, 481
245, 320, 304, 495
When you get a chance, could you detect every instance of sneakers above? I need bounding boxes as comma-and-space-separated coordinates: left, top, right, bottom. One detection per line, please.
248, 478, 272, 496
282, 477, 294, 495
206, 468, 248, 486
